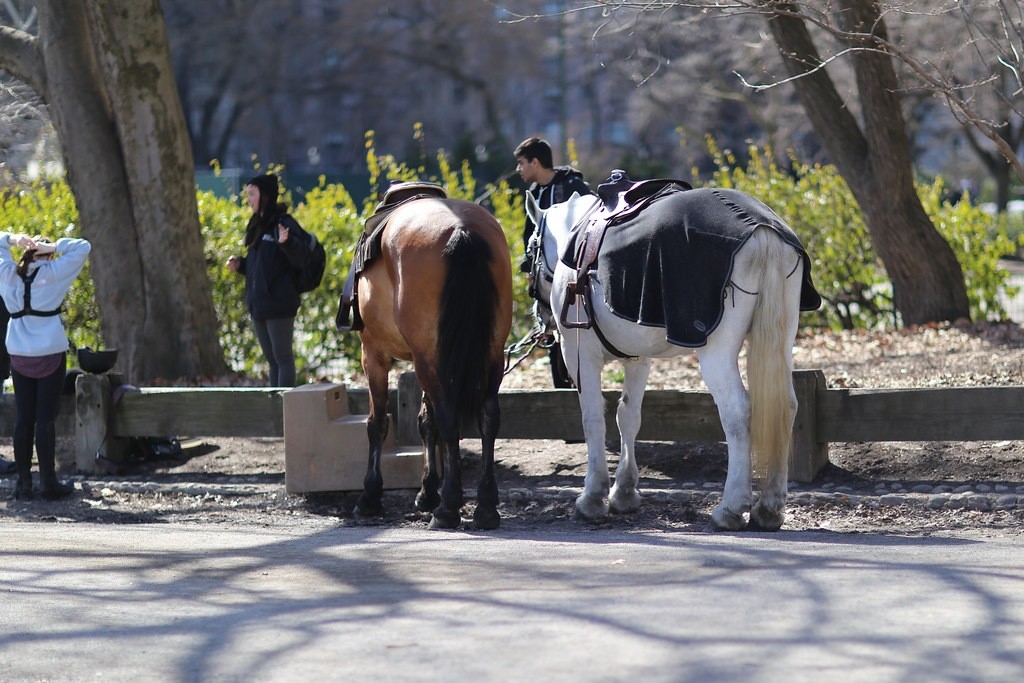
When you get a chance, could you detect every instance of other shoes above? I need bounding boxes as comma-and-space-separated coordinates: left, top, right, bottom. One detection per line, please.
40, 475, 74, 499
14, 471, 33, 501
0, 459, 16, 474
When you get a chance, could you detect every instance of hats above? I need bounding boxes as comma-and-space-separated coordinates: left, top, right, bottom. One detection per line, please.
248, 173, 279, 208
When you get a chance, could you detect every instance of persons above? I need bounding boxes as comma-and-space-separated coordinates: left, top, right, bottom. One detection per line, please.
514, 138, 599, 442
0, 231, 91, 500
226, 172, 327, 388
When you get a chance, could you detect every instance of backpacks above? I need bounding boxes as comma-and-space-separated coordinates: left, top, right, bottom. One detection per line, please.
289, 212, 326, 291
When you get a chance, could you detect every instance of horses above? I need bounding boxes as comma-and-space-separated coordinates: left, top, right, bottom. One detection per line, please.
352, 197, 513, 532
525, 190, 804, 534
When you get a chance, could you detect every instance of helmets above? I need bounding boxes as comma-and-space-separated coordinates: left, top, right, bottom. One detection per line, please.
76, 347, 118, 373
